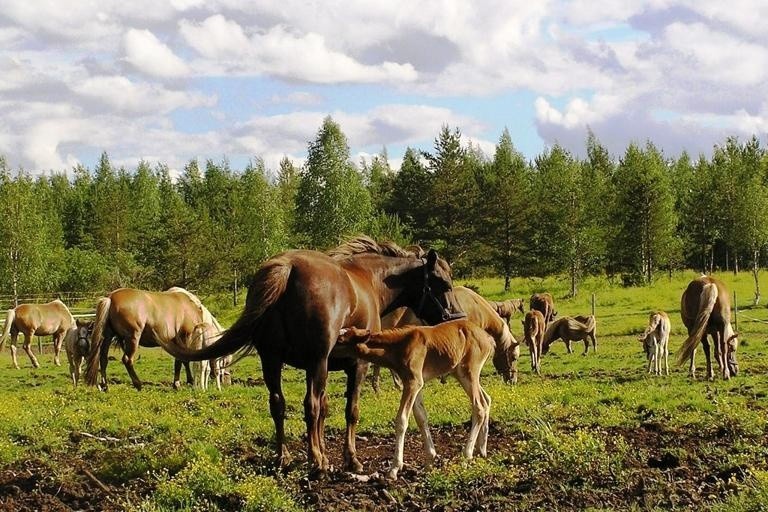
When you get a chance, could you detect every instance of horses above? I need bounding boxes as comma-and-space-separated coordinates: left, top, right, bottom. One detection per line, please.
334, 316, 498, 487
637, 310, 672, 377
0, 297, 78, 369
63, 287, 203, 395
149, 232, 467, 483
441, 284, 598, 388
167, 285, 234, 393
675, 275, 740, 382
